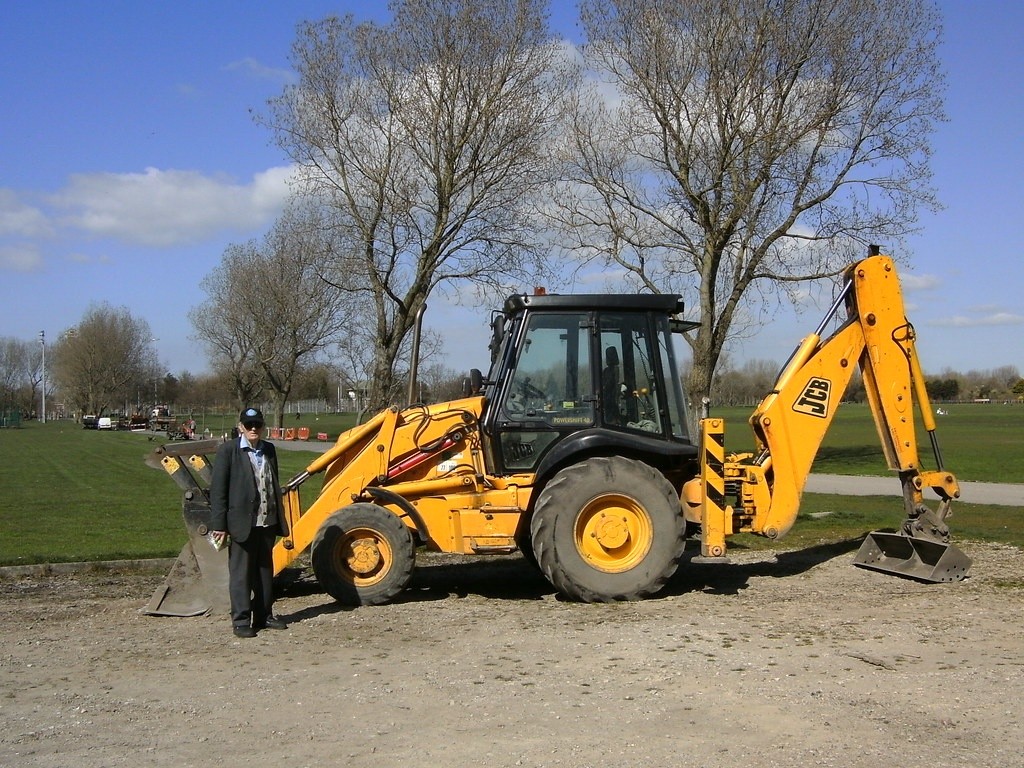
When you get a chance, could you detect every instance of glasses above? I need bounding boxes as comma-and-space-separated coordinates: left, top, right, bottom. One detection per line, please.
241, 421, 264, 430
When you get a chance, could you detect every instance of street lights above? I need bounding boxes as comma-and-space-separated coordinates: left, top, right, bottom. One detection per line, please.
38, 330, 46, 425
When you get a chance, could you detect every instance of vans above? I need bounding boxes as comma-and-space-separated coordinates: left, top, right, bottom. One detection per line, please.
98, 417, 111, 430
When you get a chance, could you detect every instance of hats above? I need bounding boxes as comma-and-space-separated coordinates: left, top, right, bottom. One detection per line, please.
240, 408, 263, 424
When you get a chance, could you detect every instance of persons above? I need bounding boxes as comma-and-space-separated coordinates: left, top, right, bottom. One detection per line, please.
181, 419, 197, 435
211, 408, 290, 638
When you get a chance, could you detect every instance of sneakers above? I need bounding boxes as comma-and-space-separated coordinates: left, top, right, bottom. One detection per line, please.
252, 618, 287, 630
233, 626, 258, 638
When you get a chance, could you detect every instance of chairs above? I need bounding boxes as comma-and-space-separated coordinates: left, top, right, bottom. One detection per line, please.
602, 345, 621, 425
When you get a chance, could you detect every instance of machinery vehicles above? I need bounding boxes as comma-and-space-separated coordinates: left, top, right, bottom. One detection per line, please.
136, 242, 974, 618
82, 405, 197, 440
